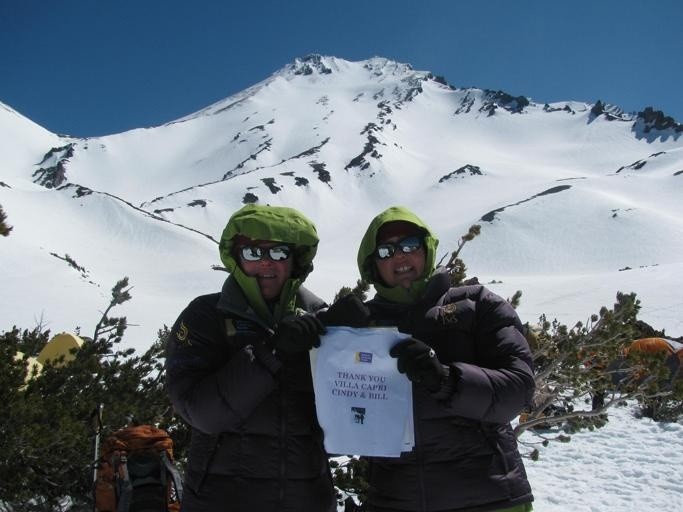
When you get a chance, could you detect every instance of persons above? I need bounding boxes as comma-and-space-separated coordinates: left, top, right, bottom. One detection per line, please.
165, 204, 338, 511
328, 207, 535, 512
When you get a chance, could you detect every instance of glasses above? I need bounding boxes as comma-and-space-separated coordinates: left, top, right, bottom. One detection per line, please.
378, 236, 423, 261
236, 244, 291, 262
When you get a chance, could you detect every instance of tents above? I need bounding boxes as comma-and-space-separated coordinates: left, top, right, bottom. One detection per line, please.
516, 334, 681, 425
10, 349, 42, 388
36, 330, 85, 368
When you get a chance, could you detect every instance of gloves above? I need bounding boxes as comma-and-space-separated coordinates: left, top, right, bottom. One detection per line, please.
270, 312, 327, 360
389, 338, 443, 392
